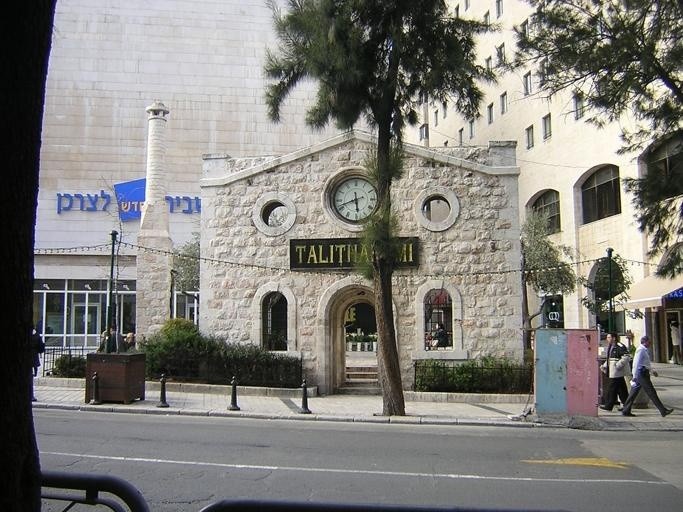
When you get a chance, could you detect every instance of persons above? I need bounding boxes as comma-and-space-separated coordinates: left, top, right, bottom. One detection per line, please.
600, 333, 632, 410
123, 332, 137, 350
31, 324, 45, 402
430, 324, 449, 347
620, 334, 673, 417
97, 324, 125, 353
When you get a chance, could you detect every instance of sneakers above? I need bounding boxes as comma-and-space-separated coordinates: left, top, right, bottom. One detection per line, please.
618, 408, 623, 411
662, 408, 673, 417
600, 405, 612, 411
625, 412, 636, 416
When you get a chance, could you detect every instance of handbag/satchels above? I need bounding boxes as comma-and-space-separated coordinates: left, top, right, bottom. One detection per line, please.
38, 334, 45, 353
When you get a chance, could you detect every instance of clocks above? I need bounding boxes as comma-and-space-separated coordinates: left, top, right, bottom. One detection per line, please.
333, 179, 378, 223
